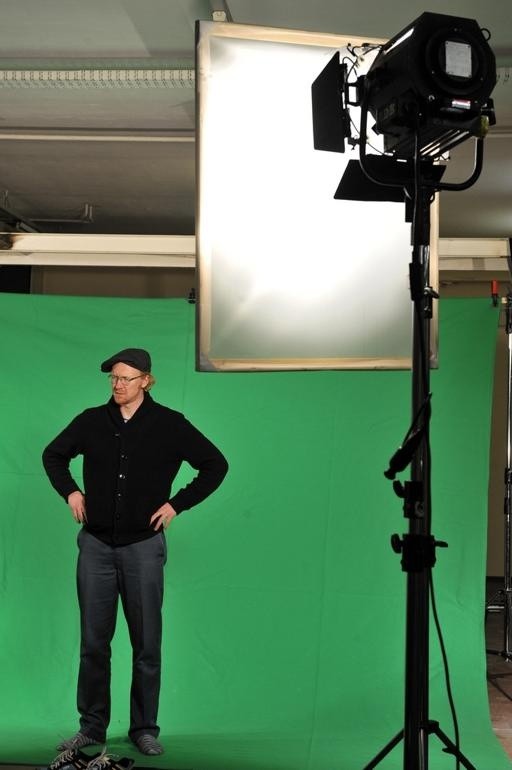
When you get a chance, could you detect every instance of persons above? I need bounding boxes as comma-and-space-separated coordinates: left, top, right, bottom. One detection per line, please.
41, 348, 229, 757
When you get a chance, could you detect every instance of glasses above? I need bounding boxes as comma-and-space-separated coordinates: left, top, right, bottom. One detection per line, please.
107, 374, 145, 386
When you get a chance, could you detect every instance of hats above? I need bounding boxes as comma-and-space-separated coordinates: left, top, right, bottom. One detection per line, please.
101, 348, 151, 372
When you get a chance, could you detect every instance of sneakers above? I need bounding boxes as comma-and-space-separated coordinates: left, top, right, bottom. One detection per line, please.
47, 739, 102, 769
84, 746, 134, 770
